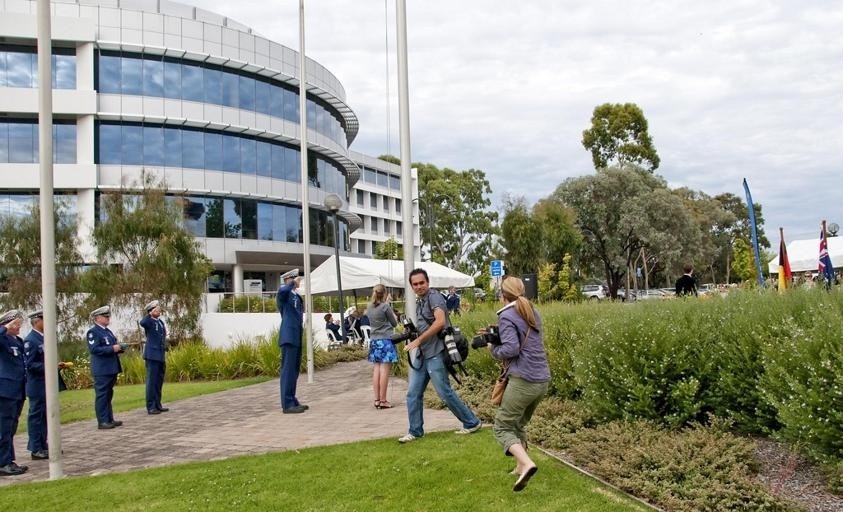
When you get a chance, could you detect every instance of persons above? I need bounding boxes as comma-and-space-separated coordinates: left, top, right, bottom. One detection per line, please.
140, 299, 169, 414
802, 271, 843, 288
477, 276, 551, 492
398, 269, 482, 443
676, 262, 698, 298
0, 309, 28, 476
366, 284, 394, 410
87, 304, 129, 429
23, 309, 69, 460
276, 267, 309, 413
323, 286, 460, 345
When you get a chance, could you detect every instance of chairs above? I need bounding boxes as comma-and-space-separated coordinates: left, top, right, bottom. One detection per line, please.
325, 325, 371, 351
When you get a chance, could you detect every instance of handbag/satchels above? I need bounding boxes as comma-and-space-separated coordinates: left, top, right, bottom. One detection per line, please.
444, 333, 468, 363
492, 369, 508, 405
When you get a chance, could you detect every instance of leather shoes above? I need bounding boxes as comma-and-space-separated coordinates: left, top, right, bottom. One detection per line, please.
283, 405, 308, 413
32, 451, 63, 459
0, 464, 28, 476
99, 421, 123, 429
148, 408, 168, 415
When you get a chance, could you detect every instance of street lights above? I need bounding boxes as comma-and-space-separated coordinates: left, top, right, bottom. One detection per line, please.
412, 193, 456, 262
827, 223, 839, 236
324, 193, 347, 346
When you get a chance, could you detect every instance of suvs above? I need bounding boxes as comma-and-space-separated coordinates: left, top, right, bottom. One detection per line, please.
578, 284, 608, 303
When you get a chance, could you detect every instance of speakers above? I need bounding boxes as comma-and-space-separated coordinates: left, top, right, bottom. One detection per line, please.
522, 274, 538, 302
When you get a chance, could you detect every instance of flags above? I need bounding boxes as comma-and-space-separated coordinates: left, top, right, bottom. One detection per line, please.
778, 228, 792, 295
818, 221, 834, 290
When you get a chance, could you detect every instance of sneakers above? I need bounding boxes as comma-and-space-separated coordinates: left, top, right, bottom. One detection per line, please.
454, 422, 482, 434
399, 433, 423, 443
514, 466, 538, 491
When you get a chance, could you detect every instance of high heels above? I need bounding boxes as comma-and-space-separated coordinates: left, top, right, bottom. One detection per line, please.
374, 399, 394, 409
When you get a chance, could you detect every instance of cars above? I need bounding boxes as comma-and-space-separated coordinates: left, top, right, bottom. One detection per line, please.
454, 287, 499, 304
615, 283, 739, 303
761, 276, 828, 292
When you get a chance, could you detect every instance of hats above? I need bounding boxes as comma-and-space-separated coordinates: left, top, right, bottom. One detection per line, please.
280, 269, 300, 280
1, 310, 18, 323
92, 306, 109, 317
28, 311, 42, 319
144, 300, 159, 311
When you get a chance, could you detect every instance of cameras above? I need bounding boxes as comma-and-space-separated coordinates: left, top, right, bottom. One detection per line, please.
472, 325, 501, 349
390, 317, 422, 345
